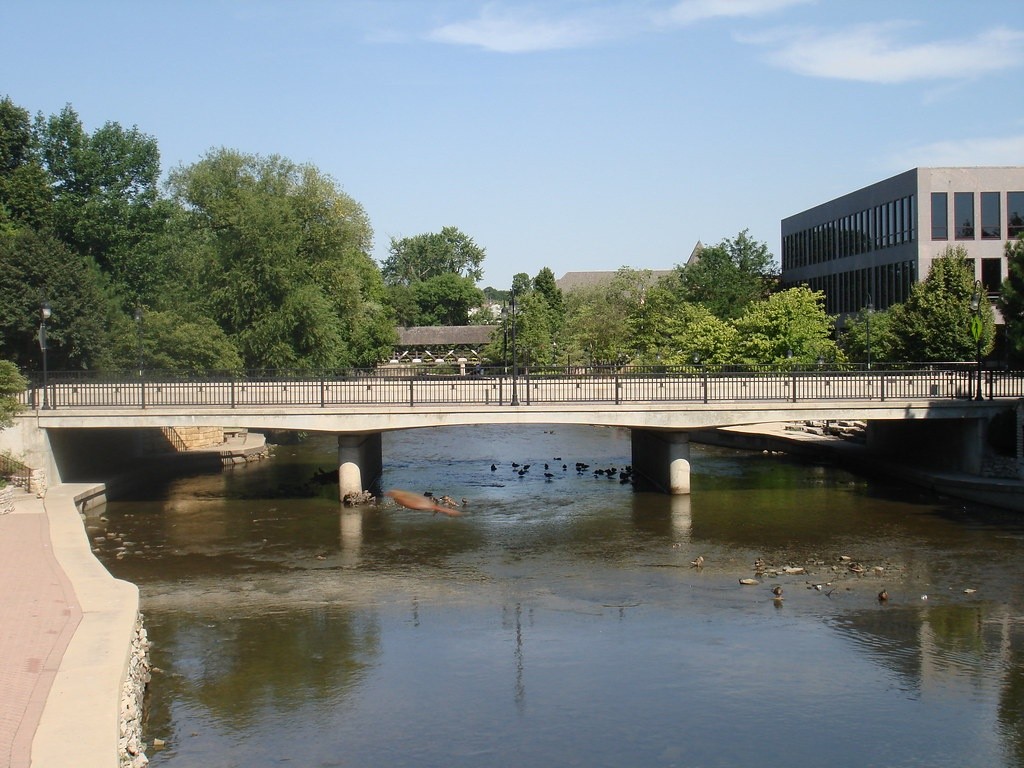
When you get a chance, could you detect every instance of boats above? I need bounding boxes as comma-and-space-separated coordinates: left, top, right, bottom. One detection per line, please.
379, 488, 466, 518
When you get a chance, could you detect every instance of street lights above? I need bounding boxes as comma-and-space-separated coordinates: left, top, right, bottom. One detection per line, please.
970, 279, 984, 400
38, 302, 53, 410
500, 294, 510, 381
507, 286, 522, 407
867, 293, 874, 386
134, 310, 148, 409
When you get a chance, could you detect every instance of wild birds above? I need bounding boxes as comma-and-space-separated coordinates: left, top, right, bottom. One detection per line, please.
691, 556, 704, 568
382, 490, 467, 516
772, 586, 784, 598
553, 457, 633, 481
512, 462, 520, 467
518, 469, 525, 475
878, 588, 889, 601
491, 464, 497, 470
755, 558, 765, 571
544, 473, 554, 479
523, 465, 530, 471
545, 463, 548, 469
